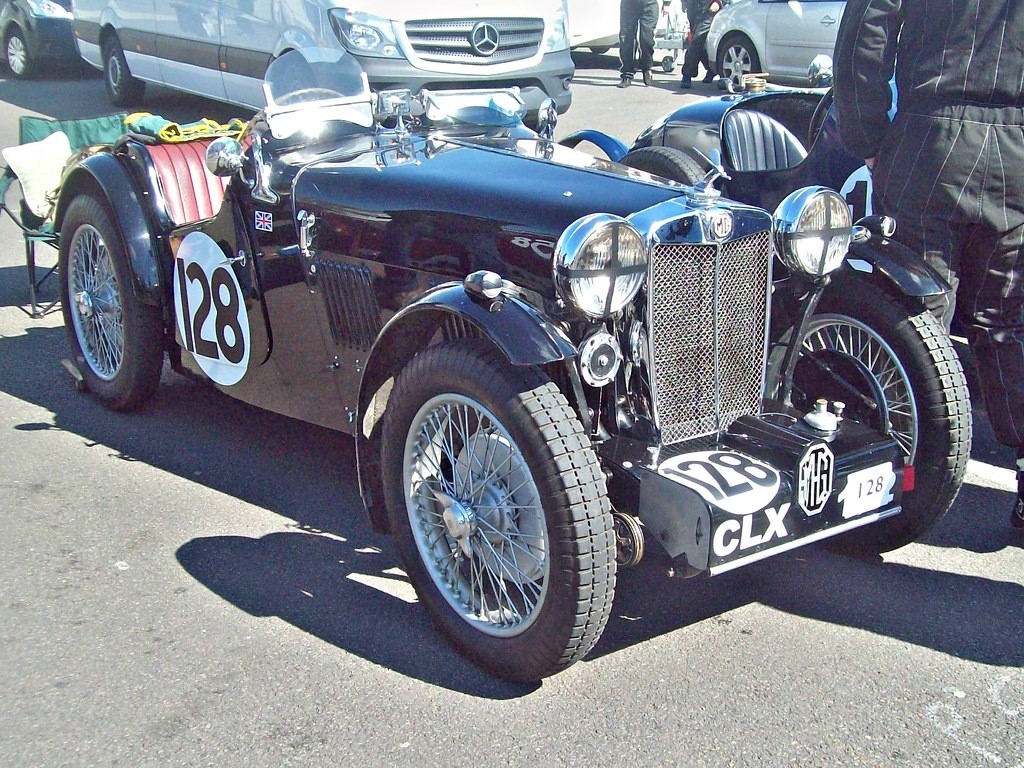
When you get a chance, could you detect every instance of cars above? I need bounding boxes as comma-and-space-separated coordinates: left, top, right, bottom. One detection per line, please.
0, 0, 79, 81
703, 1, 895, 89
46, 42, 971, 685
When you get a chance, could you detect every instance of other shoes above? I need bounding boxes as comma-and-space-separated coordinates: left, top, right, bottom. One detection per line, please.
1010, 469, 1024, 529
681, 73, 691, 88
617, 77, 631, 88
702, 70, 717, 83
643, 70, 653, 86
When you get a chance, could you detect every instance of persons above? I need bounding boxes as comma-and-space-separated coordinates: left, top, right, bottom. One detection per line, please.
679, 0, 733, 89
618, 0, 672, 88
832, 0, 1024, 529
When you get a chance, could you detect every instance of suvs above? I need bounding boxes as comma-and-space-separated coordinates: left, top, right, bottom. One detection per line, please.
69, 0, 575, 139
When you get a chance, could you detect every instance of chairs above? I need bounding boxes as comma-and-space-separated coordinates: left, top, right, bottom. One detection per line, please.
114, 126, 253, 232
0, 111, 130, 318
722, 109, 808, 171
